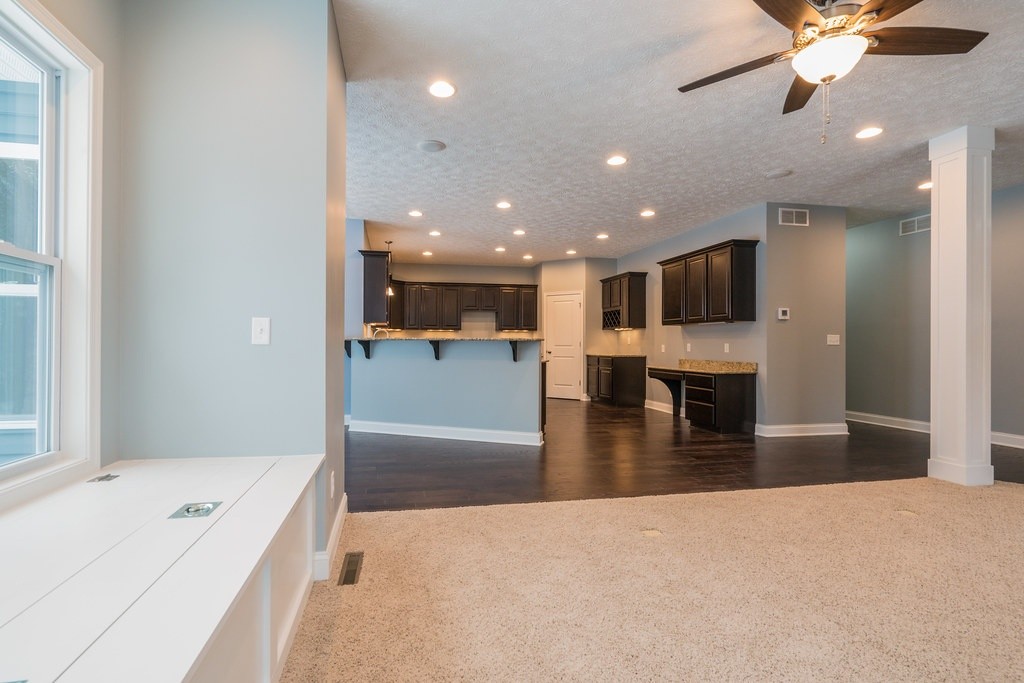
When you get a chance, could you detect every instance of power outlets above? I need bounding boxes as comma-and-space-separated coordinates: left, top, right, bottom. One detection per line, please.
251, 316, 271, 346
686, 344, 691, 352
627, 337, 630, 344
724, 343, 729, 353
661, 344, 665, 352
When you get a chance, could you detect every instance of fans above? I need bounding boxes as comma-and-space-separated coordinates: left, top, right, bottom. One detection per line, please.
678, 0, 989, 114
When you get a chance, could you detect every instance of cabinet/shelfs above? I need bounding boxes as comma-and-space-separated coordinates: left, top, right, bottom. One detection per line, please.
620, 276, 646, 328
461, 287, 499, 312
495, 286, 537, 331
420, 285, 461, 330
662, 260, 685, 326
646, 365, 757, 435
586, 355, 646, 407
685, 246, 756, 324
601, 279, 621, 309
403, 284, 420, 330
363, 255, 403, 330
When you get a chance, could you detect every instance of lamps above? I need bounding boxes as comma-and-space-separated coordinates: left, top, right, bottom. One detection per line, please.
791, 34, 869, 85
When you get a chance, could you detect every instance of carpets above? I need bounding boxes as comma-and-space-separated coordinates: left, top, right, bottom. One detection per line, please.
278, 477, 1024, 683
